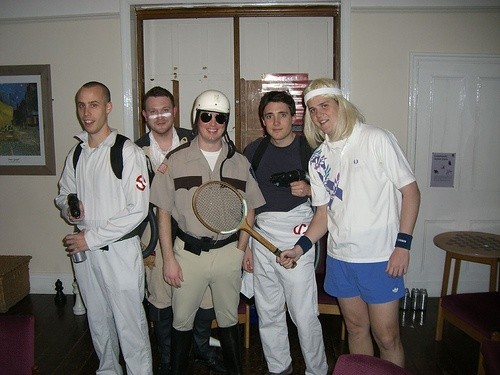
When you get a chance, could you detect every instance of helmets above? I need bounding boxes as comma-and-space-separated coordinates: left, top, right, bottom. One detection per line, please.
193, 90, 230, 124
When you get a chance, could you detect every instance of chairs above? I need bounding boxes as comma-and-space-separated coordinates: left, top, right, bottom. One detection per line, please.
435, 289, 500, 375
315, 229, 346, 341
250, 239, 320, 328
211, 297, 252, 349
0, 312, 40, 375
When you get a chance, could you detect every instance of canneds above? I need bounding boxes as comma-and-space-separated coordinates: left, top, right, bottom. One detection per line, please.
402, 288, 427, 310
67, 232, 87, 263
400, 310, 425, 326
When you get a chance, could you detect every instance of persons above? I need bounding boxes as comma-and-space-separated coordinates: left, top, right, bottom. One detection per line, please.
242, 89, 330, 375
134, 86, 266, 375
53, 80, 153, 375
275, 77, 420, 367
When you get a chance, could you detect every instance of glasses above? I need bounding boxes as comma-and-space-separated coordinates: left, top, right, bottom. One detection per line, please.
199, 112, 227, 125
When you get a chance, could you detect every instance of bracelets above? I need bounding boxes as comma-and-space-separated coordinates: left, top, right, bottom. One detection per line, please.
294, 236, 313, 255
394, 232, 413, 250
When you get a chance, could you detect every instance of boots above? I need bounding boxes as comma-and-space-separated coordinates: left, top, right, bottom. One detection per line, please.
143, 297, 173, 375
191, 307, 229, 374
217, 321, 244, 375
171, 324, 193, 375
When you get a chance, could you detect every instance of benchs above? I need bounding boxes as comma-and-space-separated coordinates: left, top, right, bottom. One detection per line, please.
0, 255, 32, 312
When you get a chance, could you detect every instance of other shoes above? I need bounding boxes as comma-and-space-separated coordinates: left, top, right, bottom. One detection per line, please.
264, 363, 292, 375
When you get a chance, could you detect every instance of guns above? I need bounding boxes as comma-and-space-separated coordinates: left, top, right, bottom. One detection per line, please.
66, 192, 81, 219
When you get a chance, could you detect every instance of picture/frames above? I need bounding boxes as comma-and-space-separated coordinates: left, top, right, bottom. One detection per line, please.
0, 64, 57, 176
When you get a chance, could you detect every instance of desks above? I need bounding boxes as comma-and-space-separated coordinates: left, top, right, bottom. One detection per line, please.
433, 230, 500, 298
